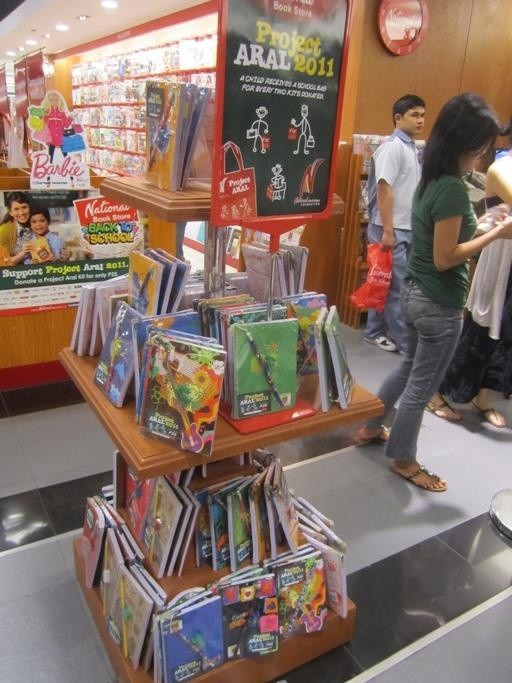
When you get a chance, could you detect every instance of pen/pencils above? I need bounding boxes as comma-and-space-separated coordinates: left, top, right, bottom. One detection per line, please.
233, 590, 259, 657
102, 541, 109, 615
138, 264, 155, 297
288, 563, 317, 633
104, 309, 126, 394
160, 350, 197, 449
118, 574, 129, 659
173, 625, 215, 668
244, 330, 287, 409
333, 326, 355, 382
144, 91, 175, 172
149, 483, 159, 563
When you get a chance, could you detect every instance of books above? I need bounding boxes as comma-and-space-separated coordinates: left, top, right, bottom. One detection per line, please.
71, 37, 218, 192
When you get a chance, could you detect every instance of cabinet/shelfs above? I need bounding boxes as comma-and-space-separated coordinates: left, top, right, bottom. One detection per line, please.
0, 0, 225, 192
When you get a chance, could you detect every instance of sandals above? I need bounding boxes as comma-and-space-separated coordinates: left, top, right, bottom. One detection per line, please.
353, 424, 388, 447
391, 462, 447, 492
425, 393, 463, 422
471, 396, 506, 428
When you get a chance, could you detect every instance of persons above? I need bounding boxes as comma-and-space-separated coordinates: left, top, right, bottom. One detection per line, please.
36, 90, 82, 182
0, 189, 64, 272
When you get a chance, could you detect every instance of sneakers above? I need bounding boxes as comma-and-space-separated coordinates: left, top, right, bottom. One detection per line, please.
362, 336, 397, 352
